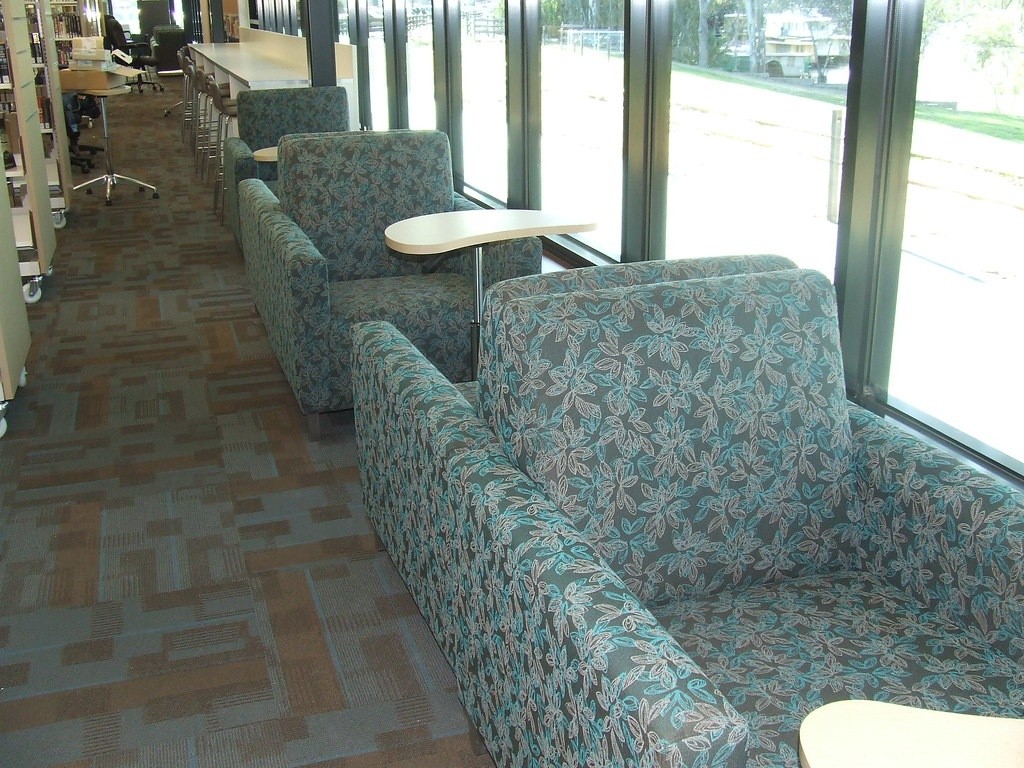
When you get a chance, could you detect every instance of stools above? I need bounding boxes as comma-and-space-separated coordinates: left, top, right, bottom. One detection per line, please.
174, 46, 238, 214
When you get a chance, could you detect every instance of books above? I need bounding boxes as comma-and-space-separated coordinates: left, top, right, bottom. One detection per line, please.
0, 10, 82, 130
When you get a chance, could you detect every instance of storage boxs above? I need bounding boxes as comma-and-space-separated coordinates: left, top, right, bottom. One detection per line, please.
72, 49, 133, 64
69, 61, 109, 71
70, 36, 103, 49
59, 66, 146, 95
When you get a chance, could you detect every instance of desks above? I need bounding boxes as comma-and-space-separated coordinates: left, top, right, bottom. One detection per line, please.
252, 145, 279, 164
383, 209, 596, 381
62, 88, 159, 203
158, 69, 183, 116
189, 42, 308, 165
792, 700, 1024, 768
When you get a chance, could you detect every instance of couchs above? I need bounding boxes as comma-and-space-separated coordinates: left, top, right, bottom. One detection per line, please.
223, 85, 350, 252
349, 252, 1024, 768
236, 127, 545, 422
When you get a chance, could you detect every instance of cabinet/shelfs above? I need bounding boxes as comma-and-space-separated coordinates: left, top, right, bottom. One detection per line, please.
0, 0, 87, 128
0, 0, 57, 304
24, 0, 73, 229
0, 156, 34, 440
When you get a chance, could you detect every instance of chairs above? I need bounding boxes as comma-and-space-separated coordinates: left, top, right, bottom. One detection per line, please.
105, 14, 164, 94
